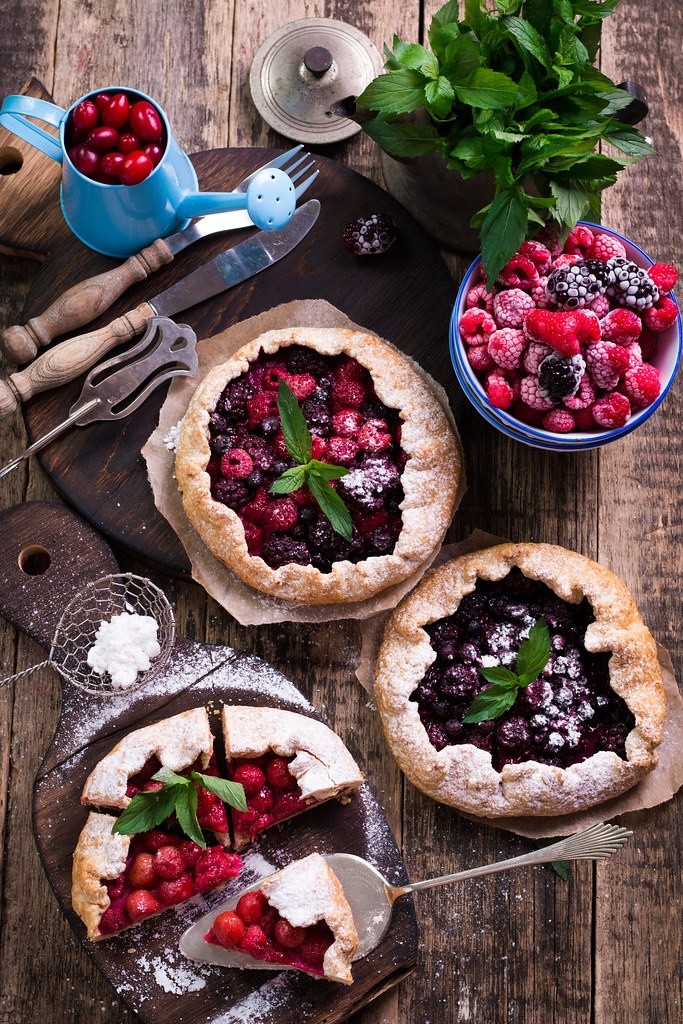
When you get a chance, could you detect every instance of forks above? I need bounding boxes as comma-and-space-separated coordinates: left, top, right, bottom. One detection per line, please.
0, 145, 319, 365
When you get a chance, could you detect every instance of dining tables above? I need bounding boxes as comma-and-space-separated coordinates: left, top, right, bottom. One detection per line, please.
0, 0, 683, 1024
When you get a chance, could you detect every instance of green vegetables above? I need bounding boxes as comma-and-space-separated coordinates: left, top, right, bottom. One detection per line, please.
353, 0, 657, 293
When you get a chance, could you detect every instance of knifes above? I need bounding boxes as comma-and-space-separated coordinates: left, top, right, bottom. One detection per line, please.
0, 198, 322, 415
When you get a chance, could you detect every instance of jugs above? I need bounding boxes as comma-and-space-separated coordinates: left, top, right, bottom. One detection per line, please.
0, 87, 297, 259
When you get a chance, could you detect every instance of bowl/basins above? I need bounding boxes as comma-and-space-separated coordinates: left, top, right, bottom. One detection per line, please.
449, 220, 683, 453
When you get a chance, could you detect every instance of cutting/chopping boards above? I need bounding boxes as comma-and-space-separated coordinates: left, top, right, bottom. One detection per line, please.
0, 501, 419, 1024
16, 148, 468, 575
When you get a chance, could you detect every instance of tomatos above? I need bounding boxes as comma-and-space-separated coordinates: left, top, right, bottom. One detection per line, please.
70, 93, 162, 186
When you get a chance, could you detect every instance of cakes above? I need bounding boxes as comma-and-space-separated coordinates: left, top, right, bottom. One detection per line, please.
174, 327, 460, 603
374, 542, 666, 817
203, 852, 359, 985
73, 703, 365, 941
